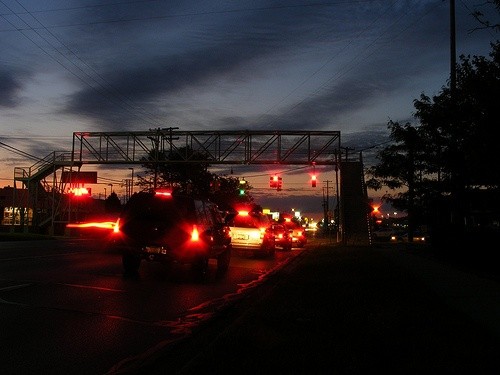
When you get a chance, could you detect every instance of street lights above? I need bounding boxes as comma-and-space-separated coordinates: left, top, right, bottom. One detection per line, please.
107, 183, 113, 195
128, 167, 134, 196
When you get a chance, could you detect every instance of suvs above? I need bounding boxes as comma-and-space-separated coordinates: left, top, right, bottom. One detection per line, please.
104, 200, 232, 282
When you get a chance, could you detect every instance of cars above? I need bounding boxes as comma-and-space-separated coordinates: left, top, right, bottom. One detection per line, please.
224, 207, 317, 256
372, 226, 430, 242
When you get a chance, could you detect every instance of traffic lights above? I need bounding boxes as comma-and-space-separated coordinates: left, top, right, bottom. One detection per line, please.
239, 180, 246, 195
277, 176, 282, 192
269, 176, 278, 187
312, 175, 317, 187
